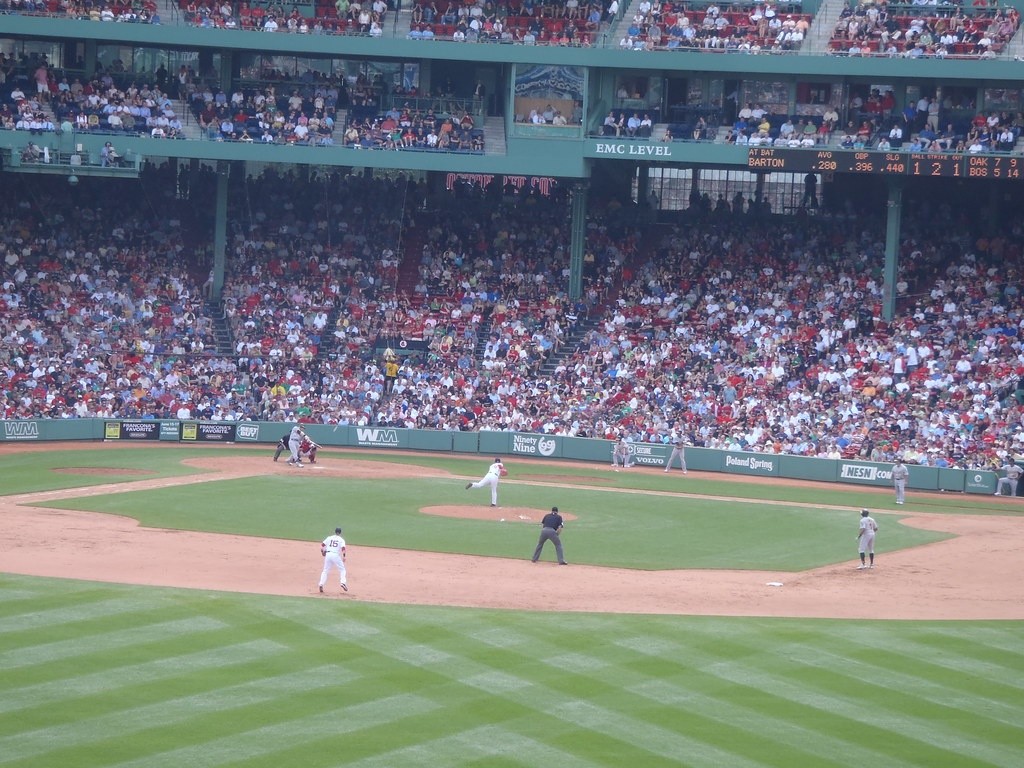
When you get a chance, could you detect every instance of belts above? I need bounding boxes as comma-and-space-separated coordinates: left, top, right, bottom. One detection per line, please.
490, 472, 498, 476
291, 439, 298, 441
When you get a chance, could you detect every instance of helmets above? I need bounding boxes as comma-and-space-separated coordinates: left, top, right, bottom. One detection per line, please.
860, 509, 869, 517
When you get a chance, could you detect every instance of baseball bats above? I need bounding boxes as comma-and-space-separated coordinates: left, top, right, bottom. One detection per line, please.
622, 447, 626, 467
304, 438, 322, 448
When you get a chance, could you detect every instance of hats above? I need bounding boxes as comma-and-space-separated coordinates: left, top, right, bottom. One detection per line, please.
495, 458, 500, 463
552, 507, 559, 512
894, 458, 902, 462
300, 423, 306, 429
676, 431, 683, 435
335, 527, 341, 533
615, 436, 621, 440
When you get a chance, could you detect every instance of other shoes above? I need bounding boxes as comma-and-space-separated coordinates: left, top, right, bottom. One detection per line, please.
559, 562, 568, 565
995, 492, 1002, 496
273, 457, 277, 461
466, 483, 472, 489
319, 586, 323, 593
310, 461, 316, 463
286, 460, 293, 465
297, 463, 304, 468
857, 565, 873, 569
896, 501, 903, 504
490, 504, 495, 507
611, 463, 618, 467
340, 584, 348, 591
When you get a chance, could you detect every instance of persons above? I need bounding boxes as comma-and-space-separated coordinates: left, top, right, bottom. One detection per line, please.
665, 430, 687, 474
889, 458, 909, 504
611, 436, 636, 467
0, 0, 1024, 471
465, 458, 503, 506
532, 507, 567, 565
299, 438, 317, 463
855, 509, 878, 569
286, 424, 310, 468
318, 528, 348, 593
994, 458, 1024, 496
273, 431, 292, 462
